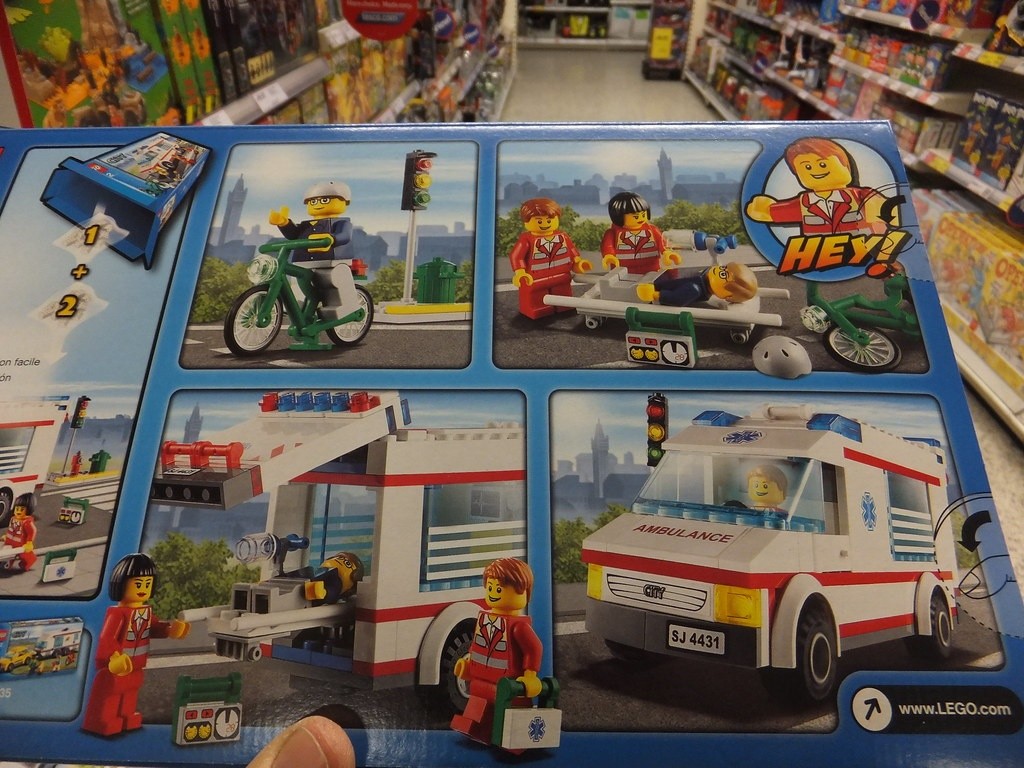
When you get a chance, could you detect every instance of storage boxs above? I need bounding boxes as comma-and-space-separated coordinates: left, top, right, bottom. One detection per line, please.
0, 1, 1024, 768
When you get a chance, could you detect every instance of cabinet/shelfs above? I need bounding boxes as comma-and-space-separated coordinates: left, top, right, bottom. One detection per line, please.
188, 54, 395, 126
515, 7, 651, 44
919, 43, 1023, 441
683, 0, 778, 121
764, 5, 974, 173
